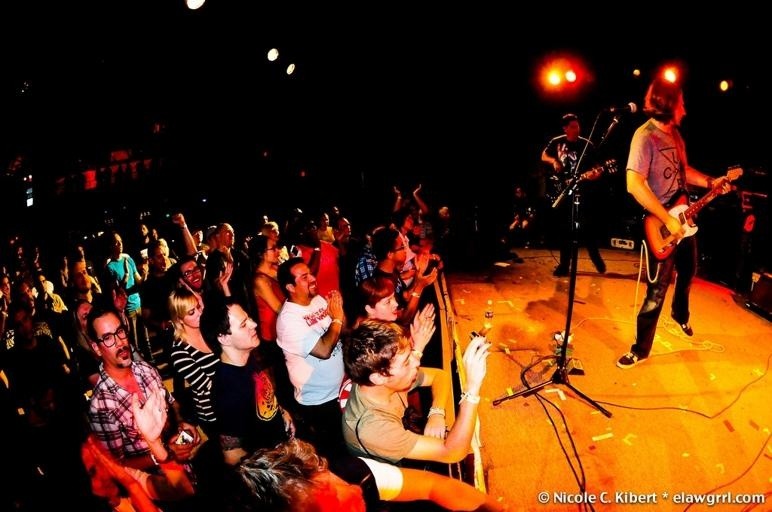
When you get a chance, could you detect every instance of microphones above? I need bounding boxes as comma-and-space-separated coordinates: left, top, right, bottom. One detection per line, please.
604, 102, 637, 138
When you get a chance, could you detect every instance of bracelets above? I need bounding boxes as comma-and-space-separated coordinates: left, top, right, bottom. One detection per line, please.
707, 176, 712, 191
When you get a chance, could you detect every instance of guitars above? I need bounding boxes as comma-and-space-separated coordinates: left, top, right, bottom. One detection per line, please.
641, 166, 744, 261
546, 159, 618, 210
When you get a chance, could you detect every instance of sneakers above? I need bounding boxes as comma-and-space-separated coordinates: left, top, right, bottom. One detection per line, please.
616, 350, 649, 369
671, 313, 694, 339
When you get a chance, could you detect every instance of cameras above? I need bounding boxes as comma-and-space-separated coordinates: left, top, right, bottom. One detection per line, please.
176, 431, 193, 445
470, 331, 481, 350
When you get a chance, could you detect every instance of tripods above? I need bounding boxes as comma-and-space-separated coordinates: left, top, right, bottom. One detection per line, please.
493, 189, 613, 419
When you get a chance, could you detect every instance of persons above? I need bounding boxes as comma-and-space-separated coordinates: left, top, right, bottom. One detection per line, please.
0, 183, 505, 512
509, 186, 534, 231
542, 113, 606, 277
615, 78, 733, 370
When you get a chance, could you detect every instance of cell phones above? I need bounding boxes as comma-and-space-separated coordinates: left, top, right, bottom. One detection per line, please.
290, 245, 302, 258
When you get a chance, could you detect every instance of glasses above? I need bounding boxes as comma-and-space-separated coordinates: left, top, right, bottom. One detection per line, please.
266, 244, 279, 252
182, 266, 201, 278
96, 324, 128, 348
